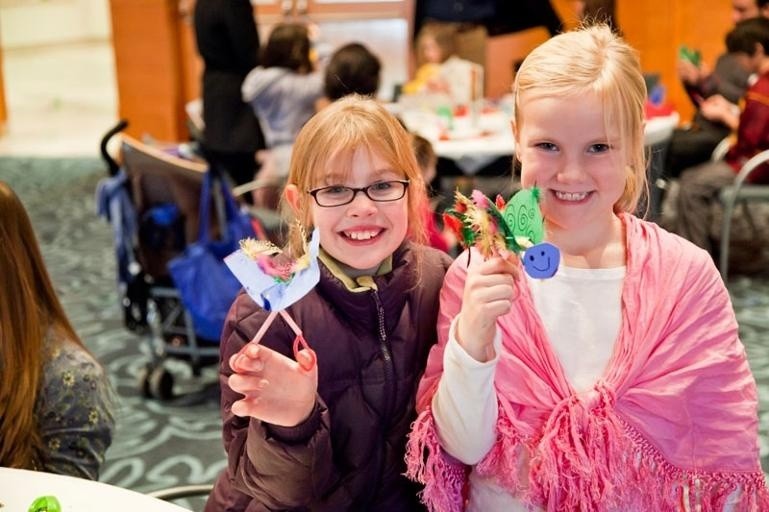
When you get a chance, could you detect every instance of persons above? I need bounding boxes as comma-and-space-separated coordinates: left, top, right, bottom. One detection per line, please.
2, 181, 114, 480
204, 95, 454, 512
404, 23, 769, 512
679, 15, 765, 255
664, 0, 769, 189
407, 23, 472, 101
316, 43, 382, 114
193, 0, 261, 204
242, 22, 326, 175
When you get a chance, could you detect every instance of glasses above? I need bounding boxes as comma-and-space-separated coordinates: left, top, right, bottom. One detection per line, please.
305, 179, 410, 207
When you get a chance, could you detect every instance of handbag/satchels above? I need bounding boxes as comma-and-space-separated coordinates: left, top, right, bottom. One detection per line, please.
168, 167, 259, 344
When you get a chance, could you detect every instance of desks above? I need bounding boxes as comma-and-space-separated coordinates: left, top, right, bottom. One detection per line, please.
380, 95, 680, 216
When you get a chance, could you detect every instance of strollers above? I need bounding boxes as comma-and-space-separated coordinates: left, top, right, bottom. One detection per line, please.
96, 118, 266, 401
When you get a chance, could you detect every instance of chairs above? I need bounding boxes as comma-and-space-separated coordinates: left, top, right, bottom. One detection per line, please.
686, 132, 769, 289
178, 141, 286, 237
184, 96, 206, 153
